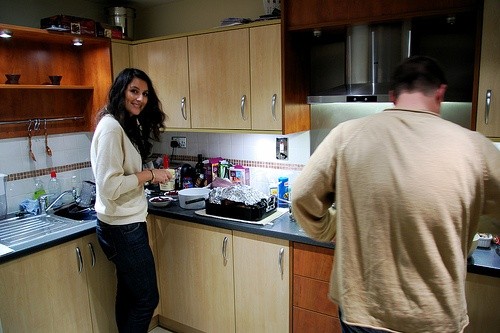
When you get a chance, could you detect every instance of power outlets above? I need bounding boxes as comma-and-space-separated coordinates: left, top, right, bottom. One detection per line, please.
173, 137, 187, 148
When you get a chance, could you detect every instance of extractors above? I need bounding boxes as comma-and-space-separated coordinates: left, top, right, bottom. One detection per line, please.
292, 8, 476, 103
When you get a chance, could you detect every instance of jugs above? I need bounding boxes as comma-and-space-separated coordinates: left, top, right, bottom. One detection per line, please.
0, 172, 8, 220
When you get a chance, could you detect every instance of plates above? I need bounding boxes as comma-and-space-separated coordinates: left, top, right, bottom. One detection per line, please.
149, 196, 173, 207
164, 191, 178, 199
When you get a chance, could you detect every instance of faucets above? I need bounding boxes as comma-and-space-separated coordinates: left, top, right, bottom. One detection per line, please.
45, 186, 77, 211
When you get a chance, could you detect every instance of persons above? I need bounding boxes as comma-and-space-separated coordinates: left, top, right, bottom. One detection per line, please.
290, 58, 500, 333
90, 68, 171, 333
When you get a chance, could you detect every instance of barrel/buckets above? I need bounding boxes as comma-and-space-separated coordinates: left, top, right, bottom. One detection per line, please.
104, 6, 136, 41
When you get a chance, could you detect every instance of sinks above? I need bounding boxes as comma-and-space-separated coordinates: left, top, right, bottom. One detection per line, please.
48, 202, 98, 223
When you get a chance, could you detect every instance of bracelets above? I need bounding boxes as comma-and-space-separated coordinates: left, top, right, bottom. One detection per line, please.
146, 170, 154, 182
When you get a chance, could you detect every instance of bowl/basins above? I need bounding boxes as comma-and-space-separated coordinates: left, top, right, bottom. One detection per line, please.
477, 233, 493, 248
4, 74, 21, 84
468, 233, 481, 260
178, 188, 210, 209
49, 76, 63, 85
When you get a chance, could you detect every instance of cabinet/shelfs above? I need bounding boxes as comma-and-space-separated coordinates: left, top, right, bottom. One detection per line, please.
467, 0, 500, 144
0, 214, 342, 333
0, 17, 311, 141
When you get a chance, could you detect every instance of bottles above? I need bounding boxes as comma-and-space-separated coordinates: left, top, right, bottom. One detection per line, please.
48, 170, 62, 208
181, 153, 232, 190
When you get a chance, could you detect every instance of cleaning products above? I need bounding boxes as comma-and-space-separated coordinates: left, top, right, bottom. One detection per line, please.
47, 171, 62, 208
33, 177, 45, 199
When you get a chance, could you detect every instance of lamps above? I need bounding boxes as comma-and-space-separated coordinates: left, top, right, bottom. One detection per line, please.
0, 29, 12, 38
73, 39, 83, 45
